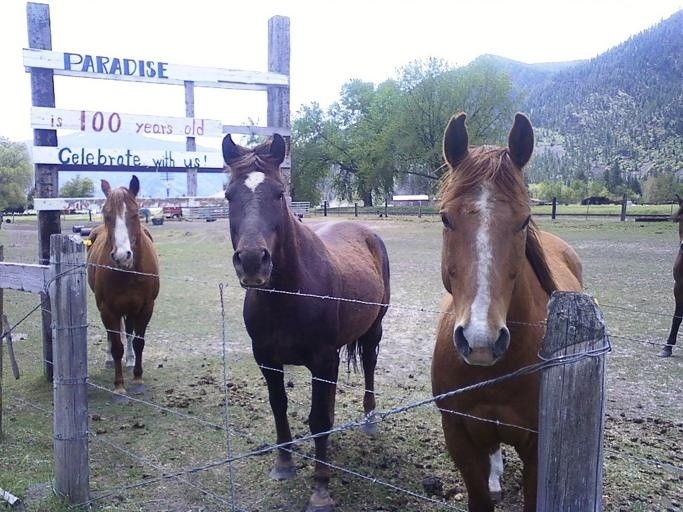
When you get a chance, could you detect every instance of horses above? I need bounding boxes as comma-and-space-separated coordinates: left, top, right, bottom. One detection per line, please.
81, 173, 162, 406
658, 194, 683, 359
425, 109, 584, 510
218, 131, 393, 512
